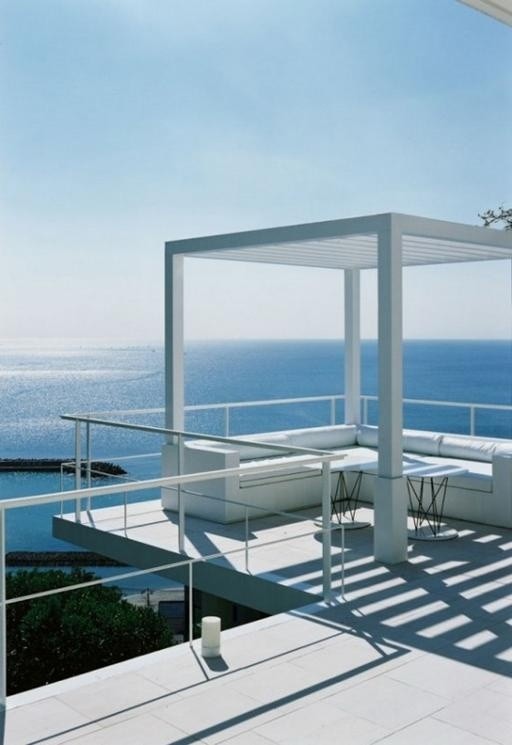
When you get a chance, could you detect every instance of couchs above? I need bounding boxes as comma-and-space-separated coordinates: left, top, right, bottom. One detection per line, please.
184, 423, 512, 530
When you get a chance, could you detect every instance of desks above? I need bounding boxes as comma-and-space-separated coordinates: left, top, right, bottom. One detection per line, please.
304, 463, 377, 531
362, 463, 468, 541
